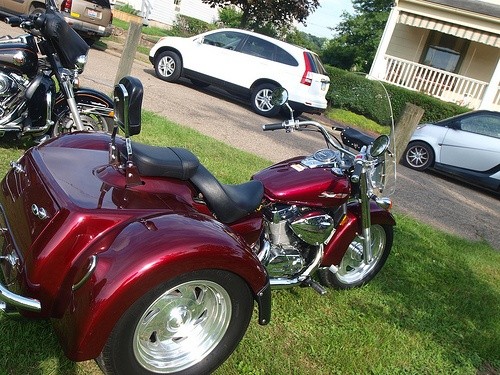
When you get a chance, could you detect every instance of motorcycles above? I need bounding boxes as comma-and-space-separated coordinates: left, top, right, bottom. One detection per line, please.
0, 73, 401, 375
1, 1, 119, 145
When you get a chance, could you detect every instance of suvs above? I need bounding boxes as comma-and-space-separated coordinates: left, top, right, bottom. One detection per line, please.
148, 26, 331, 119
0, 0, 114, 46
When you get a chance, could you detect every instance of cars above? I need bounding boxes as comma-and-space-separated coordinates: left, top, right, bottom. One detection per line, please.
403, 109, 500, 194
